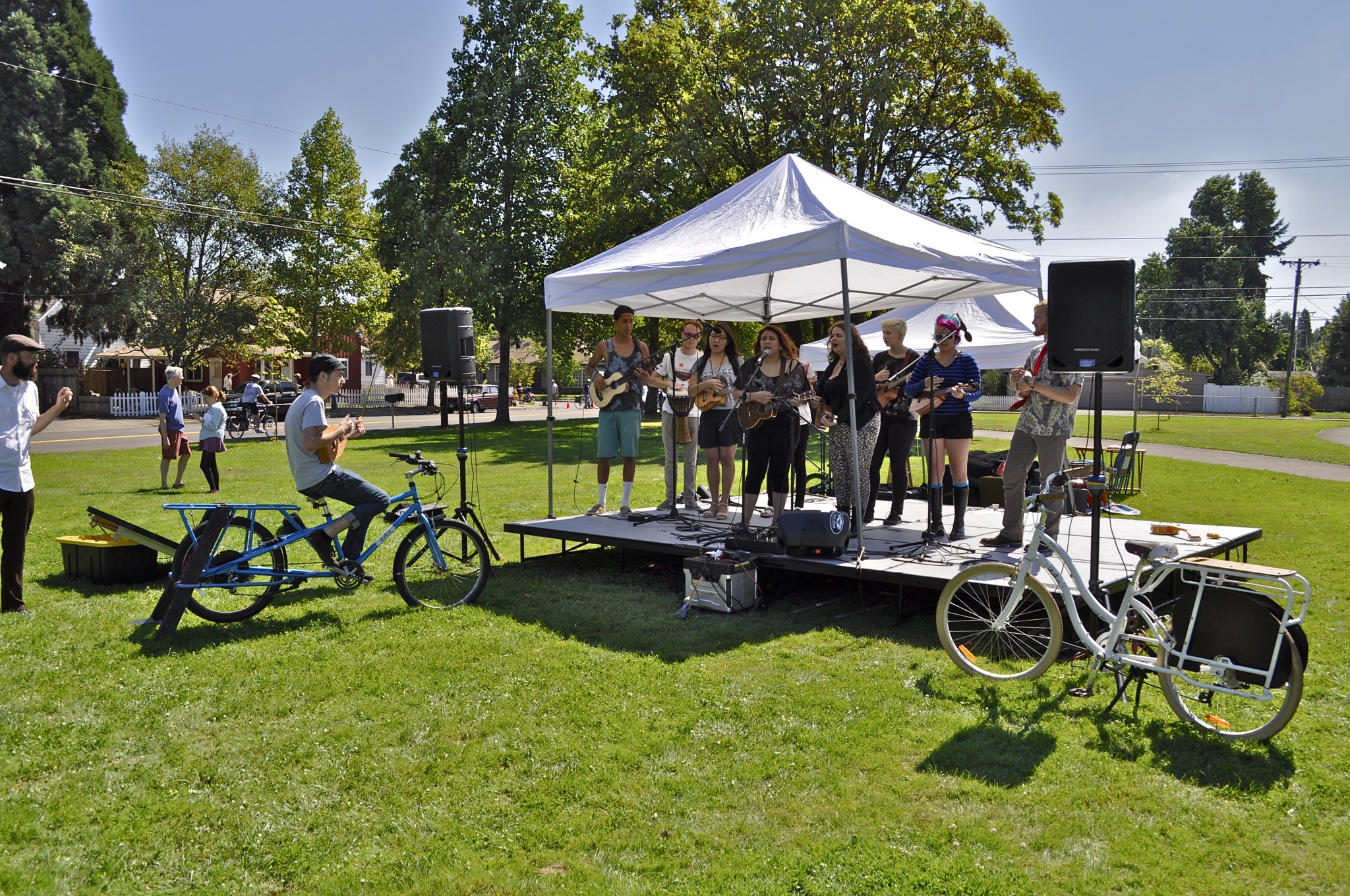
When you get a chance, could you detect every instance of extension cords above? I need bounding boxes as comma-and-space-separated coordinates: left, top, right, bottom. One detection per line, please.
721, 552, 740, 560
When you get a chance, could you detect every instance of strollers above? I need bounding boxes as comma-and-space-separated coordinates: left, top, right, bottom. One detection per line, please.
520, 387, 535, 405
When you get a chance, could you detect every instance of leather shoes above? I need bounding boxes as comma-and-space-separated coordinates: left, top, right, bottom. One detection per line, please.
979, 533, 1022, 547
1024, 539, 1052, 552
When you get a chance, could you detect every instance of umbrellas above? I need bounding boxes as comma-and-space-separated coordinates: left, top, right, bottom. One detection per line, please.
96, 339, 173, 398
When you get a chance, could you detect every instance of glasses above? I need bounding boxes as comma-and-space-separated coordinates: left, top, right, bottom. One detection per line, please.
709, 334, 728, 341
684, 332, 701, 339
14, 353, 39, 359
934, 333, 956, 340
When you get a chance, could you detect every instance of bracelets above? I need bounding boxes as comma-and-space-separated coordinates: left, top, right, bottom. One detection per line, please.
749, 392, 751, 401
593, 371, 597, 379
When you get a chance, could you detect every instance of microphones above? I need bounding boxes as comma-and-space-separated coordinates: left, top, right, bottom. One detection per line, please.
698, 319, 722, 334
757, 349, 771, 364
956, 313, 972, 342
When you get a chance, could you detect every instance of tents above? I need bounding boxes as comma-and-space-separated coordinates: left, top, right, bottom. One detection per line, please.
798, 291, 1140, 511
544, 153, 1044, 558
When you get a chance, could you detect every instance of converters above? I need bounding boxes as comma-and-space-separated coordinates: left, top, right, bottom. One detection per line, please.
628, 515, 649, 521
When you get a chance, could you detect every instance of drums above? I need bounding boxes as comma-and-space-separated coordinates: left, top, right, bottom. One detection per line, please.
666, 387, 696, 445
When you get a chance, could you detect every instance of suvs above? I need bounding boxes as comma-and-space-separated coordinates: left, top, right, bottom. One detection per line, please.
396, 371, 413, 384
398, 372, 440, 390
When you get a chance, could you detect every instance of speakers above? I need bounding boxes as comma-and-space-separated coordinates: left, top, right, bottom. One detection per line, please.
775, 509, 851, 559
419, 307, 476, 381
1047, 258, 1135, 373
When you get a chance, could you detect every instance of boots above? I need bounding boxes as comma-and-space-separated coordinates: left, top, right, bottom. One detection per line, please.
921, 484, 944, 538
833, 503, 851, 518
949, 482, 969, 540
849, 507, 865, 538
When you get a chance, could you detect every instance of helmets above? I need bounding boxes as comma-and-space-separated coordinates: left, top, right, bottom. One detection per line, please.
251, 374, 261, 379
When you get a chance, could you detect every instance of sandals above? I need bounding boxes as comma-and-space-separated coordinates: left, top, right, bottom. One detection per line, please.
587, 500, 803, 533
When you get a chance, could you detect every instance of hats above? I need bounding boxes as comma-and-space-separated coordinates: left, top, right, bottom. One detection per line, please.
0, 334, 46, 352
308, 354, 347, 372
294, 373, 301, 379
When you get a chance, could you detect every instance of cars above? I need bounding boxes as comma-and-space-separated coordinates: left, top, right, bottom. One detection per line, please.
447, 384, 516, 414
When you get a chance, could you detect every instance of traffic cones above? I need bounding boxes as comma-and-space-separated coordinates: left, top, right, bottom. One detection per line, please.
565, 396, 571, 409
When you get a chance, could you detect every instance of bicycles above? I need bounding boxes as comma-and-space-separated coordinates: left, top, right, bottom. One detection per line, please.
934, 464, 1311, 743
228, 402, 276, 439
162, 451, 491, 624
574, 393, 600, 409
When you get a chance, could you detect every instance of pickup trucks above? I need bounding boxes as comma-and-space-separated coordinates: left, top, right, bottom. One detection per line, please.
221, 379, 326, 425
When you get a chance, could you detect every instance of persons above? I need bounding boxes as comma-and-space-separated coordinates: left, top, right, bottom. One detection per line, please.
191, 386, 227, 493
292, 373, 302, 390
582, 379, 592, 409
515, 379, 523, 403
158, 366, 191, 490
302, 379, 311, 390
284, 353, 391, 581
242, 374, 273, 433
223, 371, 240, 394
552, 379, 559, 407
585, 301, 1085, 552
0, 334, 73, 614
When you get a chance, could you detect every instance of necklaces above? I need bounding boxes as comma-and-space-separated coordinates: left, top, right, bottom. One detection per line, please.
710, 353, 726, 379
940, 351, 955, 367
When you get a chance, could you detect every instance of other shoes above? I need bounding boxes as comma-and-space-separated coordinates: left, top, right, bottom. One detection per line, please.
18, 609, 35, 617
256, 429, 265, 433
864, 510, 875, 522
883, 511, 902, 525
205, 490, 219, 494
159, 483, 187, 490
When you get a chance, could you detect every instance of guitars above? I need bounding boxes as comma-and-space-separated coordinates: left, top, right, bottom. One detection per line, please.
1014, 370, 1037, 401
909, 380, 981, 417
876, 371, 914, 409
1150, 521, 1200, 538
694, 388, 734, 412
815, 402, 838, 431
317, 415, 365, 463
589, 355, 664, 409
738, 389, 816, 430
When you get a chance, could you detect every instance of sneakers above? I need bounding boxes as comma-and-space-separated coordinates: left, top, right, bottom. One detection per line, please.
308, 530, 340, 566
355, 571, 374, 580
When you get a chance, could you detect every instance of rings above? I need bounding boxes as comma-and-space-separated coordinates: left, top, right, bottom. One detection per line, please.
933, 382, 934, 384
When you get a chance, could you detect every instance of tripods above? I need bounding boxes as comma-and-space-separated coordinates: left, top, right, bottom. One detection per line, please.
405, 381, 500, 580
991, 373, 1158, 704
630, 327, 711, 531
887, 327, 976, 561
